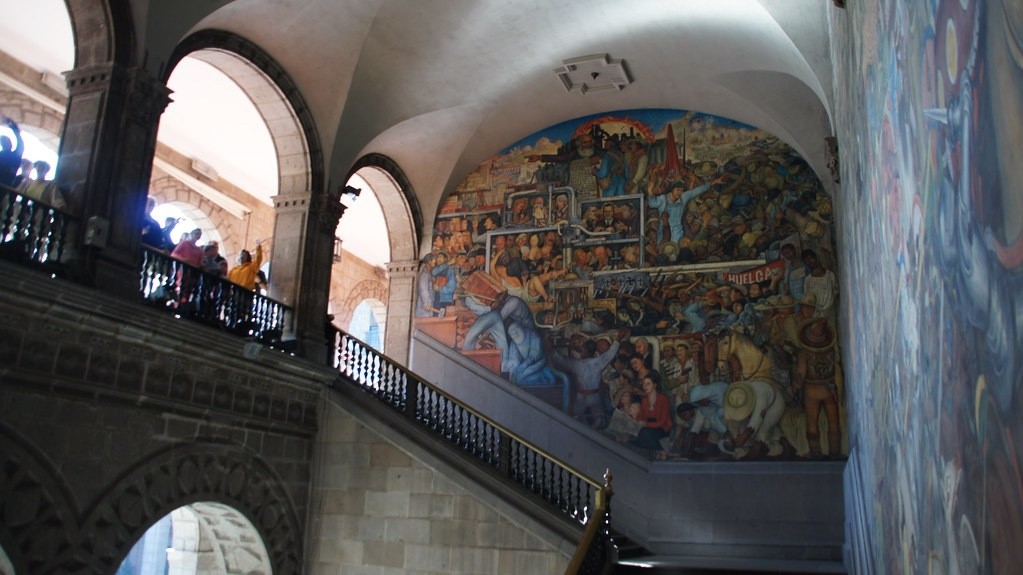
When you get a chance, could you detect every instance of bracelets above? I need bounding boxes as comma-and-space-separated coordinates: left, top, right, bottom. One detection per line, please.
258, 281, 262, 284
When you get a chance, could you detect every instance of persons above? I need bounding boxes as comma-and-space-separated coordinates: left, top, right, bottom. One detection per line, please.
0, 116, 66, 261
141, 197, 267, 328
